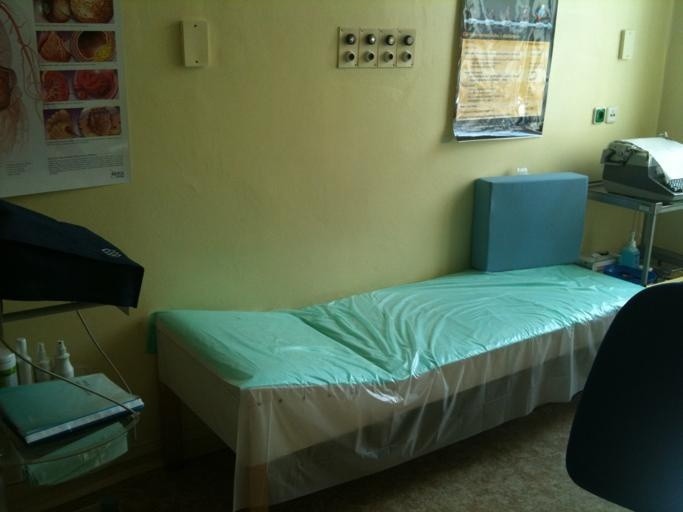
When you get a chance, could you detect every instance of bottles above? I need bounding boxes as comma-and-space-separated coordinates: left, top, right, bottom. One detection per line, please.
0, 336, 74, 387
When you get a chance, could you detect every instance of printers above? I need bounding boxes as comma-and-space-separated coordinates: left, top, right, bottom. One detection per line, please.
600, 130, 683, 204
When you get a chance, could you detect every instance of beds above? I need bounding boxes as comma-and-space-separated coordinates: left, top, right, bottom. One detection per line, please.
146, 264, 646, 512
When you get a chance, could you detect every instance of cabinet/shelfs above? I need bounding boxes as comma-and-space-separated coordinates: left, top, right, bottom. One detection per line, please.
0, 371, 161, 511
587, 177, 683, 286
